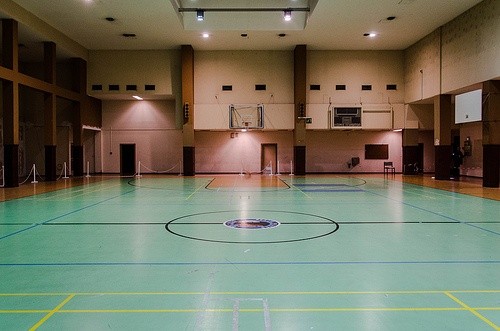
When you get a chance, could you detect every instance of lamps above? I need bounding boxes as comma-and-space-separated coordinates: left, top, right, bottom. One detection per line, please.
284, 9, 292, 22
196, 8, 205, 21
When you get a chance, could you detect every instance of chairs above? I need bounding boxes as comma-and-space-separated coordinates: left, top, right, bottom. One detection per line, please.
384, 161, 395, 175
